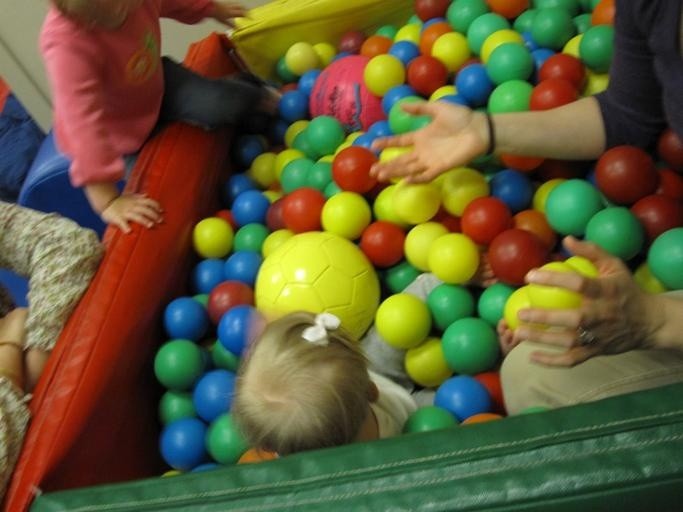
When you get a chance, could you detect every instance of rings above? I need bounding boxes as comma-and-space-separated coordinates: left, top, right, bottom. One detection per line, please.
578, 324, 593, 347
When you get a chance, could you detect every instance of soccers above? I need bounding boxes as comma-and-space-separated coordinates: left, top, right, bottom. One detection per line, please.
253, 230, 381, 344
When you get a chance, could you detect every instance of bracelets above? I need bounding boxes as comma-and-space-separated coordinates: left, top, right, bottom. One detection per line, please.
484, 115, 497, 157
0, 340, 28, 350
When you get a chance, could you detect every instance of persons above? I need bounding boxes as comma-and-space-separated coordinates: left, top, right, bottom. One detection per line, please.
233, 255, 526, 457
40, 0, 278, 236
3, 201, 107, 499
